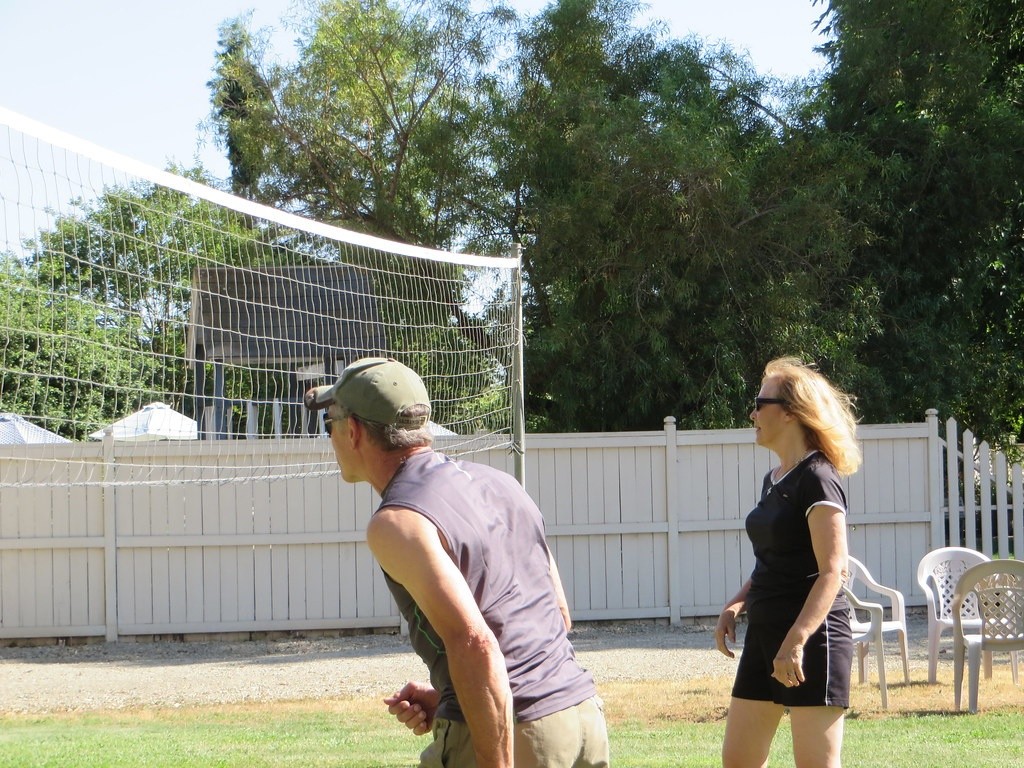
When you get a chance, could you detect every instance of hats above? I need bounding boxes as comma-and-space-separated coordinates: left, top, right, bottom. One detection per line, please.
304, 357, 432, 430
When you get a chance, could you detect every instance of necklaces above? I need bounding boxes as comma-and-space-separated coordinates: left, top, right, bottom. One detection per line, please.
767, 450, 807, 495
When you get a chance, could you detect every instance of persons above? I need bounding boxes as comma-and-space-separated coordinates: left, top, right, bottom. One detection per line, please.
304, 357, 610, 768
715, 356, 861, 768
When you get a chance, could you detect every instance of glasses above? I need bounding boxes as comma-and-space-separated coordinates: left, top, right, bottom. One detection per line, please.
754, 397, 785, 411
323, 413, 352, 438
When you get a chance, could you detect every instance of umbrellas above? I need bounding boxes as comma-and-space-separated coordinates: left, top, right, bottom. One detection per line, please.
0, 412, 72, 445
88, 401, 199, 441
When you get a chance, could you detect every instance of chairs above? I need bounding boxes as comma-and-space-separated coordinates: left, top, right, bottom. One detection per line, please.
952, 559, 1024, 715
917, 546, 1020, 685
844, 555, 911, 710
